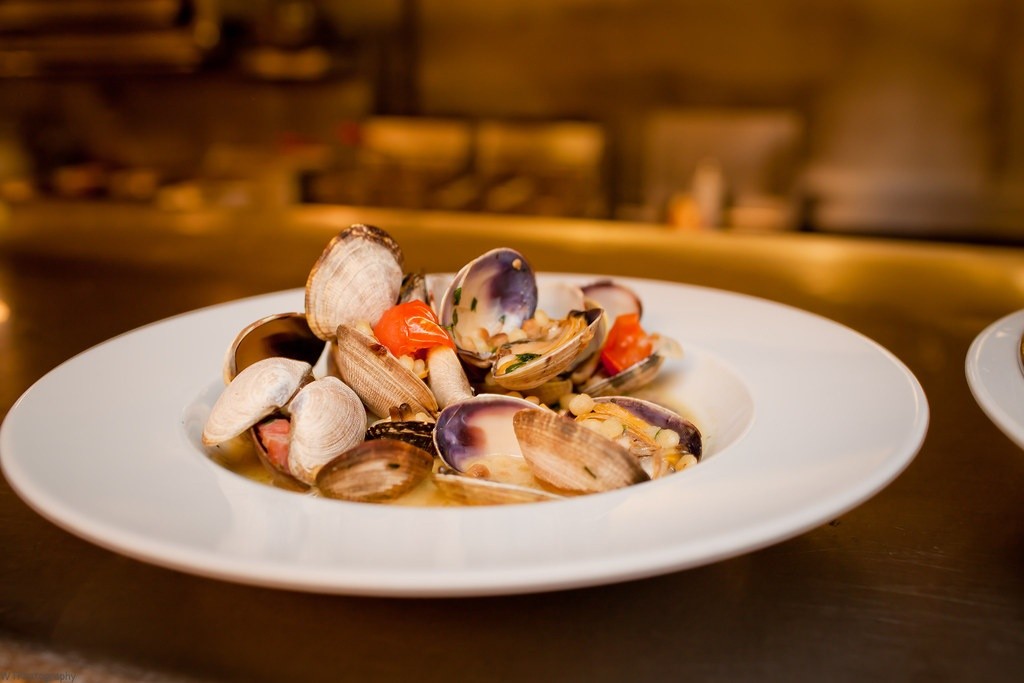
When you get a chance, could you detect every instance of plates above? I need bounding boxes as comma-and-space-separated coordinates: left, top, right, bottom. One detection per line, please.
1, 269, 931, 605
963, 310, 1024, 453
